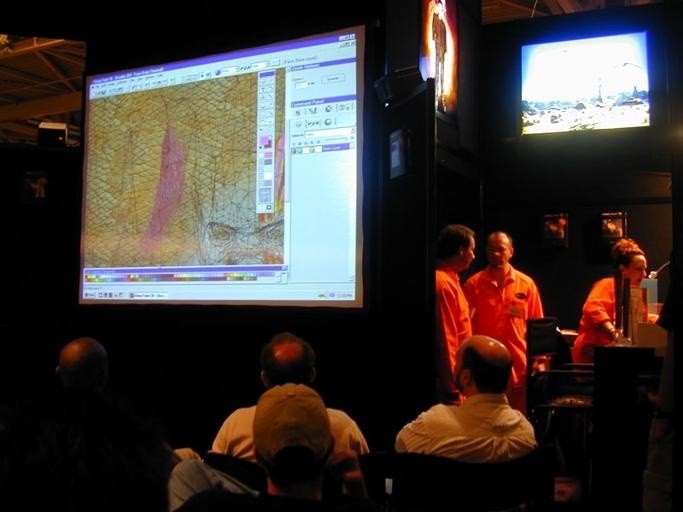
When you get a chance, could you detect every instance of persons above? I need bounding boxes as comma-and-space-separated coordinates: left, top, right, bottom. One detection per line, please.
210, 330, 370, 497
392, 334, 541, 468
569, 235, 653, 381
434, 222, 479, 401
1, 332, 161, 511
463, 227, 546, 420
167, 379, 376, 512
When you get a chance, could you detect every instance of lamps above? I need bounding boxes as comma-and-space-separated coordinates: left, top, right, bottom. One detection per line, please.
540, 211, 631, 249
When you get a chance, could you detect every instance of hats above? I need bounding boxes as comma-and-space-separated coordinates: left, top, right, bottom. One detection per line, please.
251, 382, 337, 485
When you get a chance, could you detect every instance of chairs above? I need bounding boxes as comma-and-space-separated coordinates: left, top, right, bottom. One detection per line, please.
528, 348, 664, 511
173, 444, 552, 512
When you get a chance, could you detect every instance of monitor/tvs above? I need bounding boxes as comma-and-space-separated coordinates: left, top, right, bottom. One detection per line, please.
520, 31, 652, 136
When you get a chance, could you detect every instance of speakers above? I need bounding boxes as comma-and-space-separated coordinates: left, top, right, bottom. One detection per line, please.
37, 122, 68, 144
